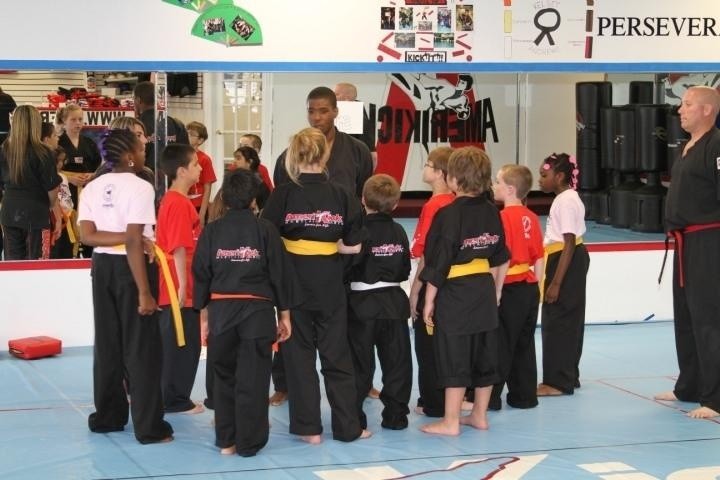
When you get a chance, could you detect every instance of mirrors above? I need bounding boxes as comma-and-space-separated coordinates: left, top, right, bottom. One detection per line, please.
0, 60, 720, 270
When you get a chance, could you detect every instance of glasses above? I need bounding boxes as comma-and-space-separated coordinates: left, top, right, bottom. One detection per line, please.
188, 132, 199, 137
425, 162, 434, 168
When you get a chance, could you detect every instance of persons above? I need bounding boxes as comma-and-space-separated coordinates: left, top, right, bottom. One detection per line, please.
654, 86, 719, 418
398, 6, 453, 29
232, 19, 250, 37
539, 153, 589, 396
79, 84, 545, 455
2, 70, 274, 264
417, 74, 473, 119
458, 9, 473, 31
335, 83, 379, 174
